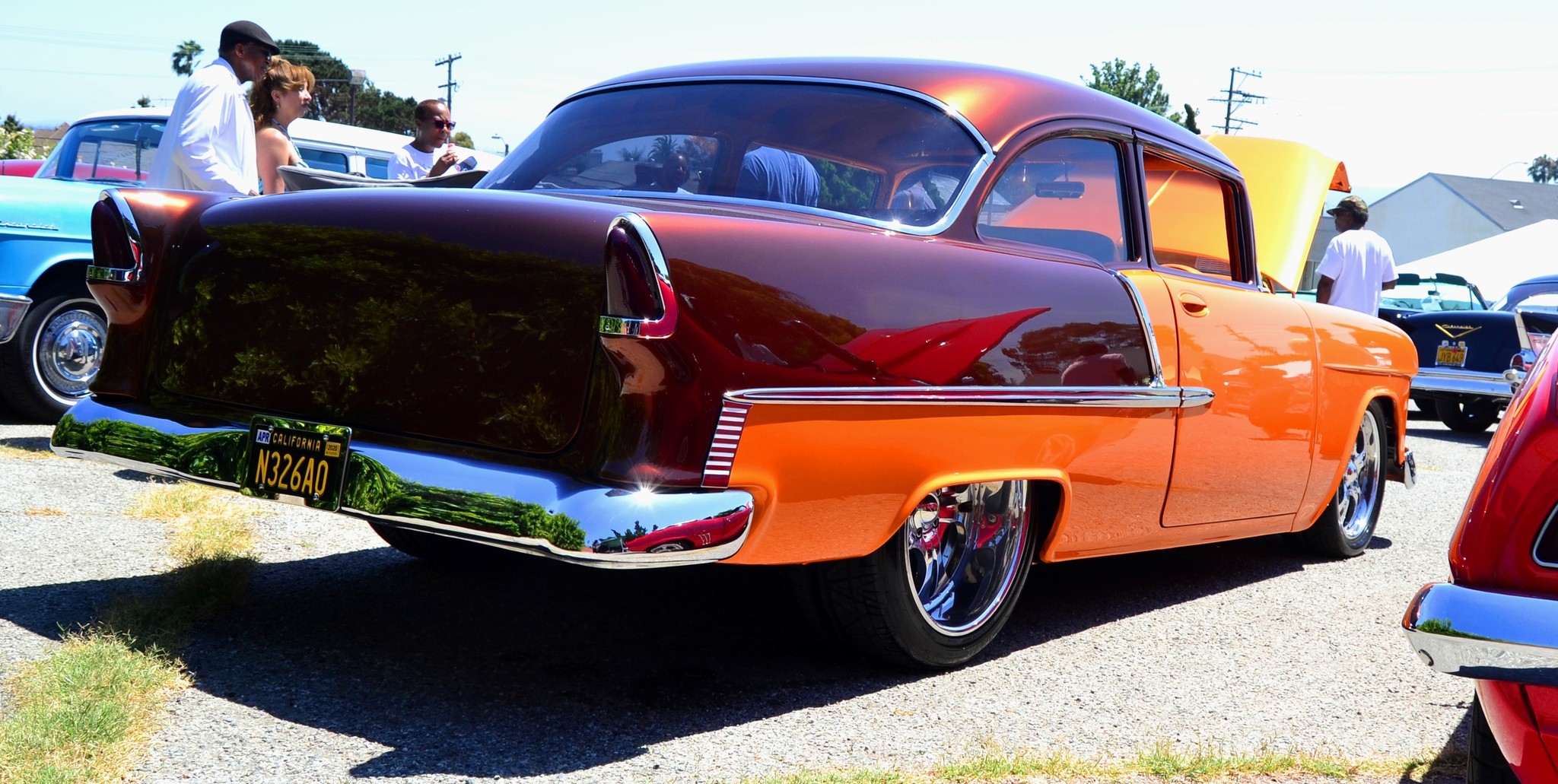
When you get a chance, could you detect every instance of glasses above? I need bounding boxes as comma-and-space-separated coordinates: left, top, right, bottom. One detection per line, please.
435, 119, 456, 131
264, 51, 272, 61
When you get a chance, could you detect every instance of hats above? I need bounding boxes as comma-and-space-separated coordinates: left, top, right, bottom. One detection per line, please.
1326, 195, 1368, 216
220, 20, 281, 55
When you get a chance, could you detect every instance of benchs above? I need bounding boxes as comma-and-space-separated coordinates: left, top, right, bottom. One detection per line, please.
845, 207, 1120, 264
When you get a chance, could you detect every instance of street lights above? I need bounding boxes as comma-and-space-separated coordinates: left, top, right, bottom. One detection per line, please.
347, 69, 367, 126
491, 133, 509, 157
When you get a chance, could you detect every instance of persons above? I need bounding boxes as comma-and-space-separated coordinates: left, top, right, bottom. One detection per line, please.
246, 56, 316, 194
385, 97, 468, 181
1315, 193, 1398, 317
891, 168, 937, 209
142, 15, 277, 200
1058, 340, 1136, 387
629, 153, 694, 193
732, 142, 820, 207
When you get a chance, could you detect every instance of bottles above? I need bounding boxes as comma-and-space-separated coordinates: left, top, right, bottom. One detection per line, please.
448, 143, 461, 165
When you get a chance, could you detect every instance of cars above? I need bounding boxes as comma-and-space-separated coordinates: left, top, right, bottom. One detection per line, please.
1273, 271, 1558, 433
1399, 324, 1558, 784
47, 56, 1417, 672
0, 106, 505, 424
